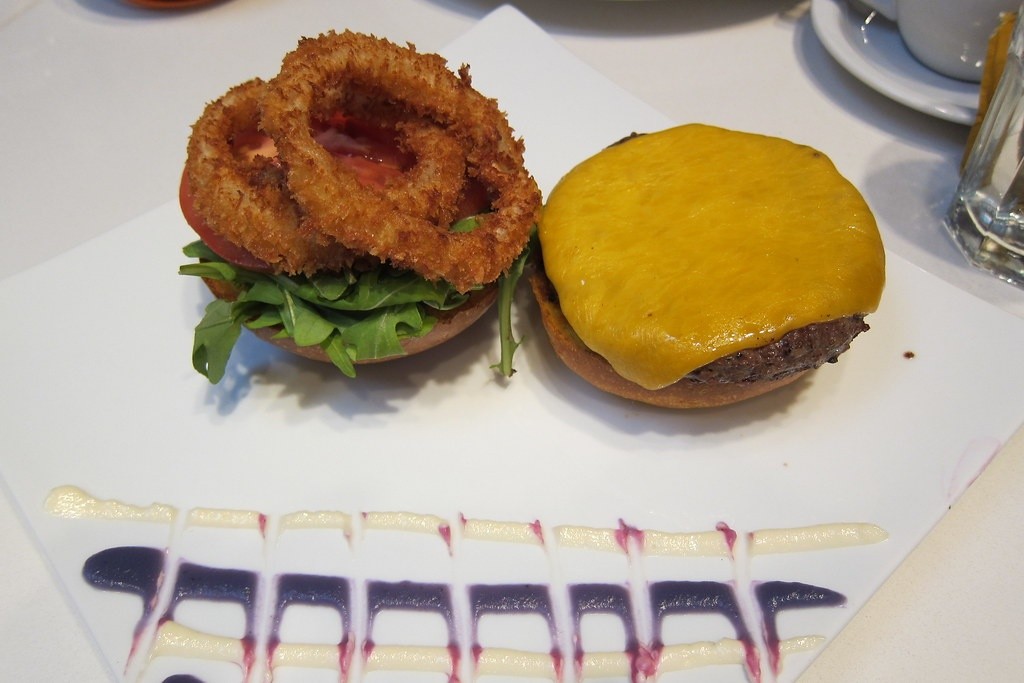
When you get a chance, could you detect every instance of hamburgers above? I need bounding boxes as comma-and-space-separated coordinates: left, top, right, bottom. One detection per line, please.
172, 30, 887, 409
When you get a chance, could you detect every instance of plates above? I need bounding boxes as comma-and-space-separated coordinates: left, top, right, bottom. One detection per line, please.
809, 0, 982, 128
1, 0, 1023, 683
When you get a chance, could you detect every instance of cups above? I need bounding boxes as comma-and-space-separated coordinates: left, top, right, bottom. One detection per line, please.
942, 8, 1024, 288
862, 0, 1024, 83
